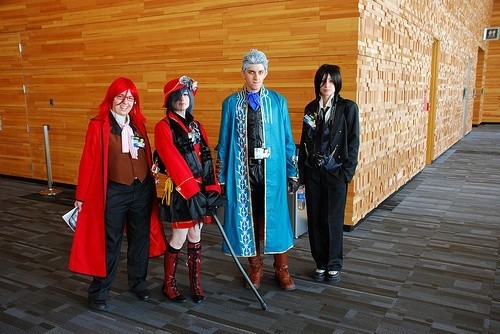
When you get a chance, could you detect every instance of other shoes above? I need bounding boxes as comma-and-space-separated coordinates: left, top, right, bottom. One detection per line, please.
315, 268, 326, 275
328, 271, 339, 277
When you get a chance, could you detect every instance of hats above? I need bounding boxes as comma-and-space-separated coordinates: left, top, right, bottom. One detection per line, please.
163, 75, 198, 108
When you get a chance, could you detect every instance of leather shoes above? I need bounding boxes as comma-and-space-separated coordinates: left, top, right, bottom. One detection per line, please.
131, 288, 152, 301
88, 295, 106, 309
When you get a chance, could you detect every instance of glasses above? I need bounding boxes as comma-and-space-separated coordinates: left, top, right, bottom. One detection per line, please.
115, 94, 134, 102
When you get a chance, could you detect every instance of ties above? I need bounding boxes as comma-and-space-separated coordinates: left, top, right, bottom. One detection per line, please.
114, 111, 138, 160
321, 106, 330, 124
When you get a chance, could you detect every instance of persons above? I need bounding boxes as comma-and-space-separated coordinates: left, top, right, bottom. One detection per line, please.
154, 75, 222, 304
215, 49, 299, 291
69, 77, 166, 309
298, 64, 360, 283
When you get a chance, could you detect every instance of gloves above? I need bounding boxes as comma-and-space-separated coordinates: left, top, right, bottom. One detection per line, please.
221, 185, 226, 196
289, 177, 299, 192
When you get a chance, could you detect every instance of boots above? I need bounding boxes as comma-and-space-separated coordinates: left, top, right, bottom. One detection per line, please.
187, 239, 206, 302
245, 249, 263, 289
273, 252, 296, 290
162, 243, 187, 303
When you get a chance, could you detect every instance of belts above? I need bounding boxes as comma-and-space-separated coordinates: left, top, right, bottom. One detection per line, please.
248, 157, 264, 165
